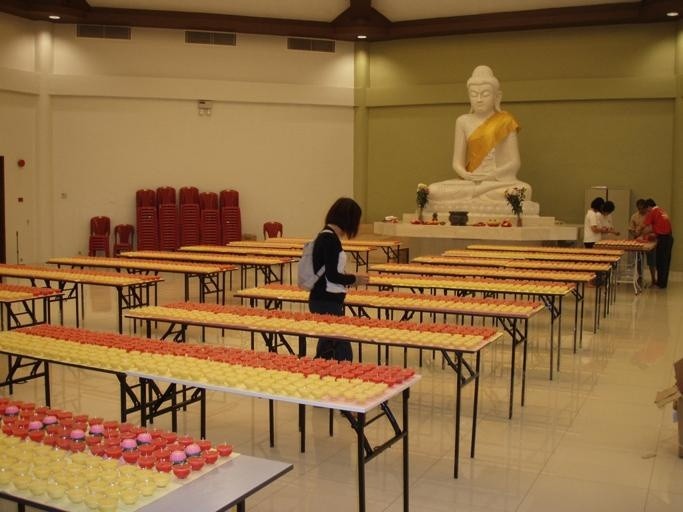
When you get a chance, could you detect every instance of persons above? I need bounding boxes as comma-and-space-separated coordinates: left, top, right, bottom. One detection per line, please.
638, 198, 673, 291
306, 195, 371, 420
582, 197, 611, 251
600, 201, 621, 240
627, 198, 657, 287
422, 64, 542, 217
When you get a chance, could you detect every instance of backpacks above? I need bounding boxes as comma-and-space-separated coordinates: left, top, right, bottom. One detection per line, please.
296, 242, 316, 291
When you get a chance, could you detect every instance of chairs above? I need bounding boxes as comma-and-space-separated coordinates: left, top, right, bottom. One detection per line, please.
82, 181, 284, 259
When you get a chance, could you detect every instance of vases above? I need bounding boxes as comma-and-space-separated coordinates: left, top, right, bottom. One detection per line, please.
515, 213, 521, 226
416, 208, 424, 220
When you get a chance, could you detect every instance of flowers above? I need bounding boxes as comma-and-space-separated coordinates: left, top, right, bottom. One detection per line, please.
504, 186, 528, 214
415, 182, 429, 205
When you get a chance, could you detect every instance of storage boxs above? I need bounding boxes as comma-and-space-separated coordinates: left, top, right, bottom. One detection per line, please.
654, 357, 682, 463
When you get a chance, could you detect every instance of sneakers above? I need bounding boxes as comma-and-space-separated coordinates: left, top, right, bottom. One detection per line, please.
636, 277, 668, 290
